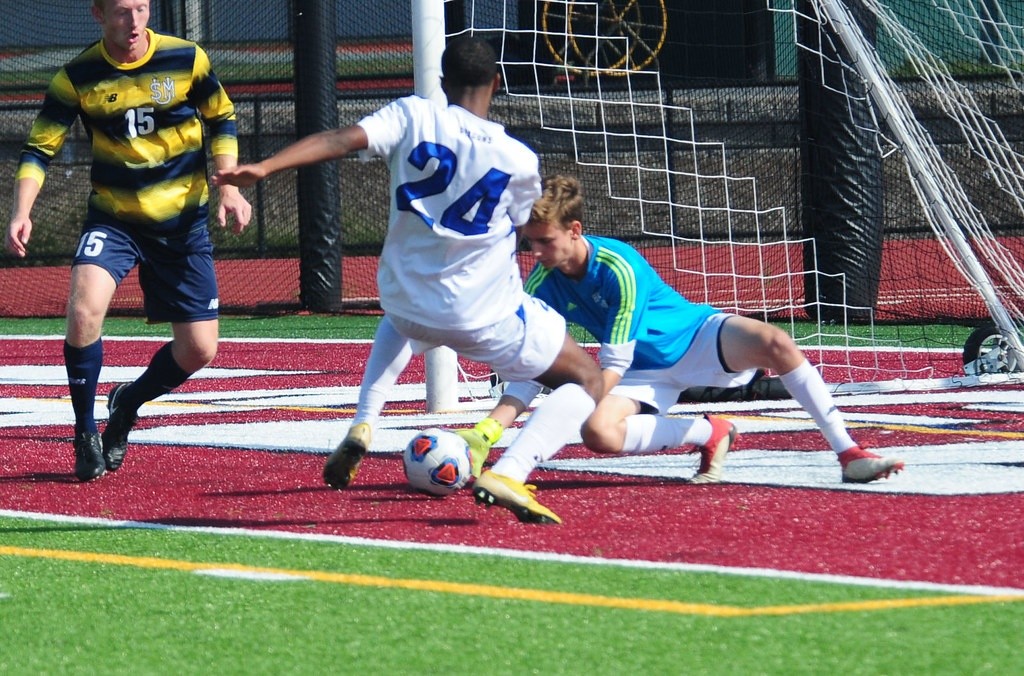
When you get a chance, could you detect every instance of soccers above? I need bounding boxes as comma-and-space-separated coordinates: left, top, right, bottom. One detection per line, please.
401, 427, 474, 496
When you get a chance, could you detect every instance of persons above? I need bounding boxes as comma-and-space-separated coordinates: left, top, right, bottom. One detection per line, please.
454, 176, 906, 488
5, 0, 252, 482
212, 35, 605, 523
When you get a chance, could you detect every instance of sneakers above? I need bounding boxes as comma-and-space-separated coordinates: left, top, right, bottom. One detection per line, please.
686, 411, 736, 484
72, 424, 106, 482
470, 470, 563, 525
105, 381, 139, 472
321, 423, 372, 487
836, 445, 904, 483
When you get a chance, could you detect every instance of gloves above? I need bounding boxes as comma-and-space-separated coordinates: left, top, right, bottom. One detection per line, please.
447, 417, 503, 481
